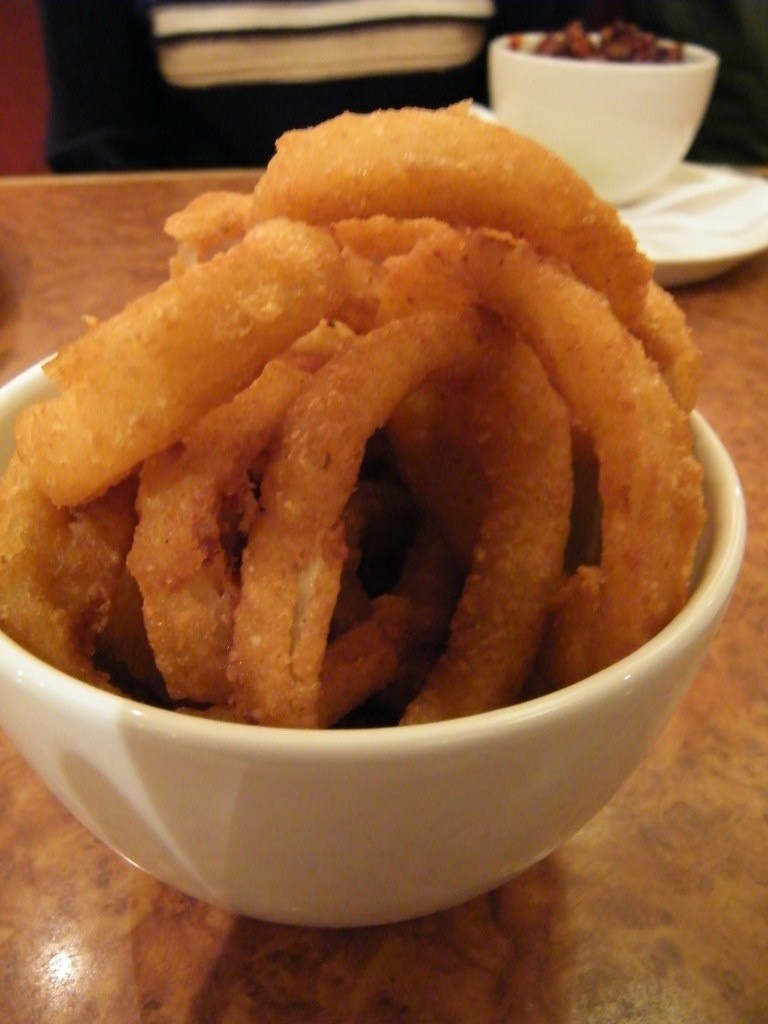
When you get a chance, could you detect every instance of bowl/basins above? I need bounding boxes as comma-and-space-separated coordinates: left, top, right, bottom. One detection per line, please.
0, 349, 748, 930
488, 32, 719, 206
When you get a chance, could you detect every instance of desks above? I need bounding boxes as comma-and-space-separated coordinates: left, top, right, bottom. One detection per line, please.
1, 170, 768, 1024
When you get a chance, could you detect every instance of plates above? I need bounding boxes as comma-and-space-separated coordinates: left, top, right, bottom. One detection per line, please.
617, 161, 768, 286
